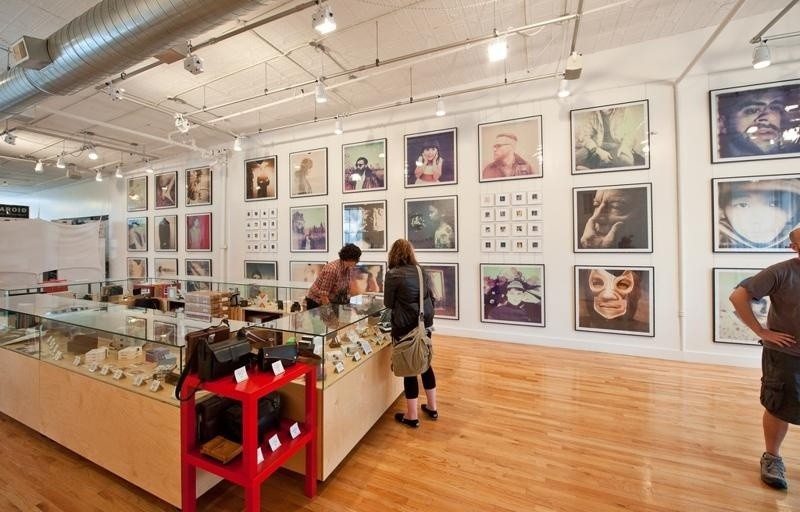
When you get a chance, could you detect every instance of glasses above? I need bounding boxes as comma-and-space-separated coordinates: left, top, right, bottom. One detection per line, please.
356, 164, 364, 167
493, 144, 509, 147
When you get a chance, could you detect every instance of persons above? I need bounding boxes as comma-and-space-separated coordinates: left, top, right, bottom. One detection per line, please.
249, 272, 262, 299
189, 218, 202, 248
482, 133, 532, 177
730, 227, 800, 489
488, 281, 530, 322
129, 223, 143, 250
415, 141, 443, 185
369, 266, 381, 292
580, 189, 643, 249
720, 87, 799, 156
579, 268, 648, 331
257, 168, 269, 197
156, 266, 166, 277
295, 159, 313, 193
719, 181, 800, 249
429, 202, 453, 248
306, 244, 362, 309
383, 239, 438, 427
577, 108, 644, 168
349, 158, 379, 188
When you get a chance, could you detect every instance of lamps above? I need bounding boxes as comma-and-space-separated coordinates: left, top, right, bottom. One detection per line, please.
753, 38, 771, 70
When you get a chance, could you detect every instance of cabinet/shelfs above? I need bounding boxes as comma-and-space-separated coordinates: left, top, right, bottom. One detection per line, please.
181, 362, 318, 512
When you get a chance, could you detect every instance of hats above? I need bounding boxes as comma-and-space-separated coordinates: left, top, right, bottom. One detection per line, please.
506, 280, 524, 289
495, 131, 519, 141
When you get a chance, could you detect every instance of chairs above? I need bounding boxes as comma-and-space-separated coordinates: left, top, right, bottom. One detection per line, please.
134, 298, 160, 310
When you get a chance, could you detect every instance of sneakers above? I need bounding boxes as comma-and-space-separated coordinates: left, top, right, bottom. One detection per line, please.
420, 403, 438, 419
395, 412, 419, 427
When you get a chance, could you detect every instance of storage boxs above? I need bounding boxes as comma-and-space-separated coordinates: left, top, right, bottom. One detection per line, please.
45, 291, 76, 299
183, 292, 225, 319
43, 280, 68, 293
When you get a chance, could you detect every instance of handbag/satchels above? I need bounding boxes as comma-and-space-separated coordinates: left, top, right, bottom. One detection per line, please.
197, 336, 254, 383
183, 319, 232, 375
257, 342, 298, 372
238, 327, 277, 364
195, 394, 237, 443
219, 392, 283, 444
390, 325, 433, 379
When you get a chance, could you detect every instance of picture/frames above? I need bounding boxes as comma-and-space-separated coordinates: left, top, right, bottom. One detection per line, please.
126, 165, 212, 291
569, 98, 655, 338
479, 262, 545, 328
477, 114, 543, 183
289, 126, 460, 321
244, 154, 277, 201
708, 77, 796, 348
243, 260, 278, 303
